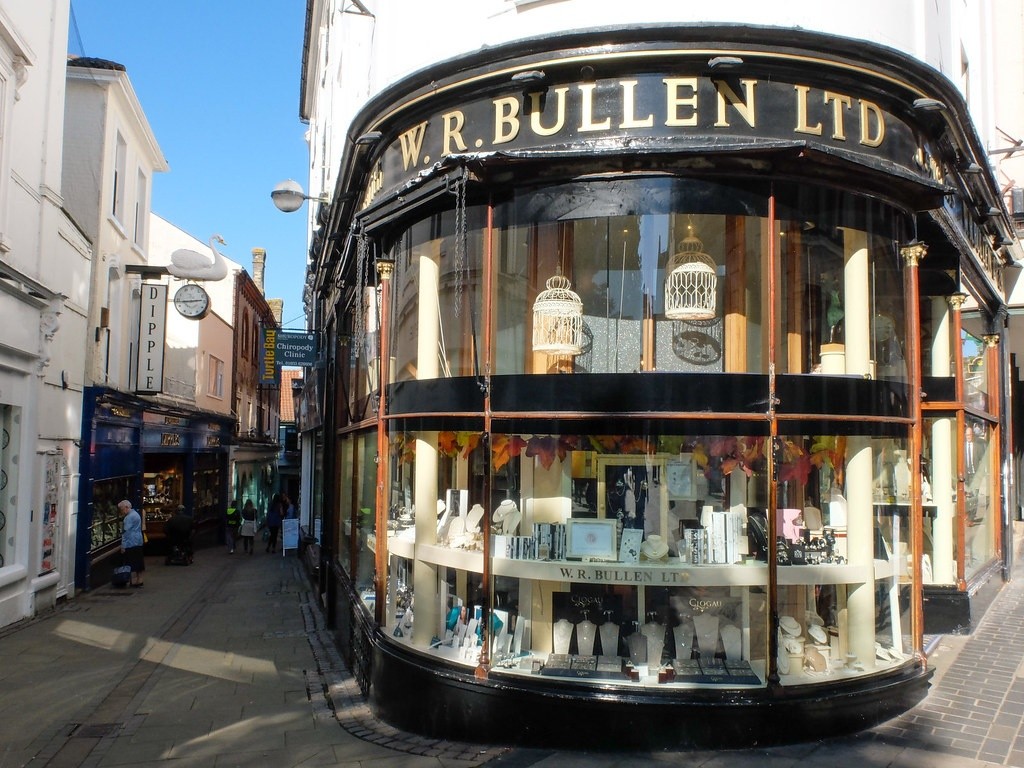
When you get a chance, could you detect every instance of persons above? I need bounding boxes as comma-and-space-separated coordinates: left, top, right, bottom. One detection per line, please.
164, 506, 195, 564
118, 500, 146, 586
963, 422, 985, 527
555, 622, 618, 642
226, 492, 298, 554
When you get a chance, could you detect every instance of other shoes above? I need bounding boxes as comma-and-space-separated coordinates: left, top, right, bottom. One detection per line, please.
265, 547, 270, 552
272, 550, 277, 553
130, 582, 142, 587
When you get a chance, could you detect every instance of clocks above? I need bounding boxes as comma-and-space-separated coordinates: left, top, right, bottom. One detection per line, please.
177, 283, 211, 322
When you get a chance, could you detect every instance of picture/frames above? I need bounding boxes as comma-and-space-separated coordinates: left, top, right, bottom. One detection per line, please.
655, 452, 698, 502
565, 518, 619, 560
597, 454, 668, 560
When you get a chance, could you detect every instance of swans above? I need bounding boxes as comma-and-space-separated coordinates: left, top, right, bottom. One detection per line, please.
165, 234, 227, 281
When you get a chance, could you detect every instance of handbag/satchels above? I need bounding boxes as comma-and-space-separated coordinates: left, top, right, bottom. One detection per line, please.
254, 510, 257, 533
112, 563, 131, 586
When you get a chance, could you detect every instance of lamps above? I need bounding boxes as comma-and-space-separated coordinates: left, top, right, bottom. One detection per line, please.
710, 55, 746, 68
316, 194, 358, 298
272, 180, 332, 212
510, 69, 547, 85
913, 98, 1023, 272
357, 130, 383, 143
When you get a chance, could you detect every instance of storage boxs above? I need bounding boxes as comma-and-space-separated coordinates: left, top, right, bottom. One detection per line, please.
377, 489, 877, 685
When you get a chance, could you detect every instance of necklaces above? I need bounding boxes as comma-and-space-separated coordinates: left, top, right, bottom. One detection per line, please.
646, 617, 739, 643
497, 503, 515, 517
646, 541, 663, 555
468, 508, 482, 522
778, 620, 825, 668
